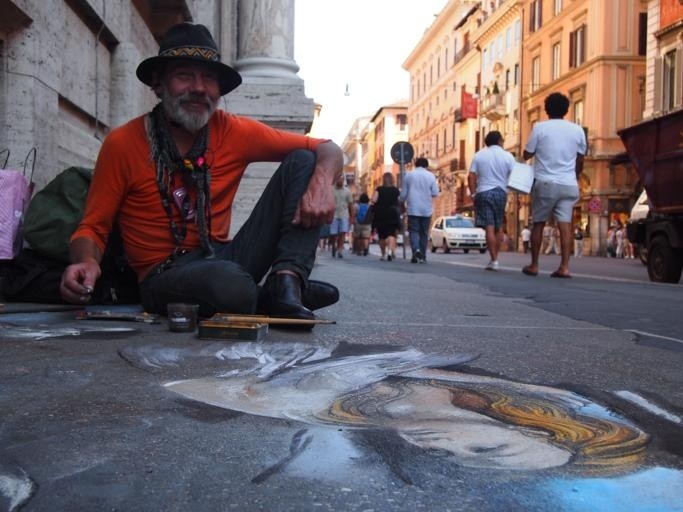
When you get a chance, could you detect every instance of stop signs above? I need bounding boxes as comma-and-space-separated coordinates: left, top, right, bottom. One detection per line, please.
589, 197, 601, 210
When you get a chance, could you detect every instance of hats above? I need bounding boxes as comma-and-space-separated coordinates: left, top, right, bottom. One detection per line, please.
136, 22, 242, 96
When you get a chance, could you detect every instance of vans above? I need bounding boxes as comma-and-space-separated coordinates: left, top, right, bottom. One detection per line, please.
627, 189, 652, 262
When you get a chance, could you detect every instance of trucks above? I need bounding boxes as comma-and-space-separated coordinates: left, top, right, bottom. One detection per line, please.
618, 105, 682, 282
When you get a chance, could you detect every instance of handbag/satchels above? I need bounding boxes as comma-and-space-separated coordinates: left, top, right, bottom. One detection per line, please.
0, 167, 140, 305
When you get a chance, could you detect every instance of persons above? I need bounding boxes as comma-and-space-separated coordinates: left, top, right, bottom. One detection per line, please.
468, 131, 516, 270
61, 24, 344, 332
523, 92, 587, 278
161, 343, 655, 481
319, 172, 406, 261
521, 225, 636, 259
399, 157, 439, 264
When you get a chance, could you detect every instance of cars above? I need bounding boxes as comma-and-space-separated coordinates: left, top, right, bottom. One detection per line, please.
372, 233, 403, 245
430, 213, 488, 255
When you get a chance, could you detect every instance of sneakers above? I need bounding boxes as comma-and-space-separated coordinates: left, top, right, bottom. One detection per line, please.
485, 260, 500, 270
380, 249, 427, 264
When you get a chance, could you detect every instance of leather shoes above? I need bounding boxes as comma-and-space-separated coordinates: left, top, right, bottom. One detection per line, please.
258, 272, 339, 332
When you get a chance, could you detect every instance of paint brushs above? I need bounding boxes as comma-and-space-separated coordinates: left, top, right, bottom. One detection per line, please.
208, 316, 336, 325
87, 316, 155, 323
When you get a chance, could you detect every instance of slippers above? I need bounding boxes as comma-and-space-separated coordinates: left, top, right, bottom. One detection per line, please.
550, 272, 573, 279
522, 265, 538, 275
